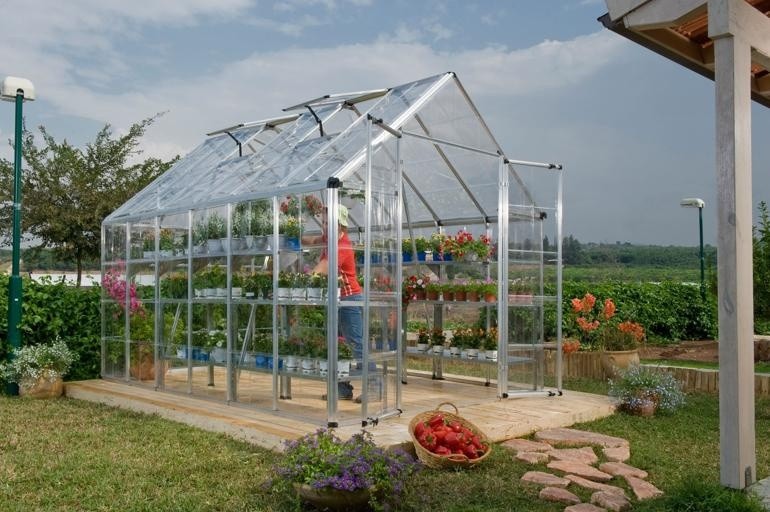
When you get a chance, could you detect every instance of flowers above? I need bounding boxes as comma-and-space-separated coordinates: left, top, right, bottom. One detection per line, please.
560, 293, 644, 354
263, 427, 423, 511
605, 358, 685, 416
101, 260, 183, 366
0, 335, 81, 389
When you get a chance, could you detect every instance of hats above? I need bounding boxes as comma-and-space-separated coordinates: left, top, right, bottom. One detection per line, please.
323, 204, 349, 227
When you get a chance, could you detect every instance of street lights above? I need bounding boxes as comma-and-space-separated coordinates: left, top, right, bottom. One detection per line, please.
678, 198, 706, 302
0, 77, 37, 396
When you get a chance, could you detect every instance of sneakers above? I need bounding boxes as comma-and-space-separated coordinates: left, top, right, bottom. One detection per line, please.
322, 382, 354, 400
356, 383, 380, 403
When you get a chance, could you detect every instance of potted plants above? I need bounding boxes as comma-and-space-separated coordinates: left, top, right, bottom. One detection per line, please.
116, 203, 533, 383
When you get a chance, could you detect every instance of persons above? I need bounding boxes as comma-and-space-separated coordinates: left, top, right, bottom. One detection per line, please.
298, 203, 385, 403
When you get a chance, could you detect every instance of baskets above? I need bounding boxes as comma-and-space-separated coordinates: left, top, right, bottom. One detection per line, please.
408, 402, 492, 471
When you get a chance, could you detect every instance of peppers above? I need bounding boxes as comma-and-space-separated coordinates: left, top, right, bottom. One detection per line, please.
415, 414, 489, 460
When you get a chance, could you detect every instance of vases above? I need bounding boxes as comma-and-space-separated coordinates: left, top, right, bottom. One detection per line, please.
635, 400, 655, 417
291, 479, 376, 506
18, 379, 64, 399
